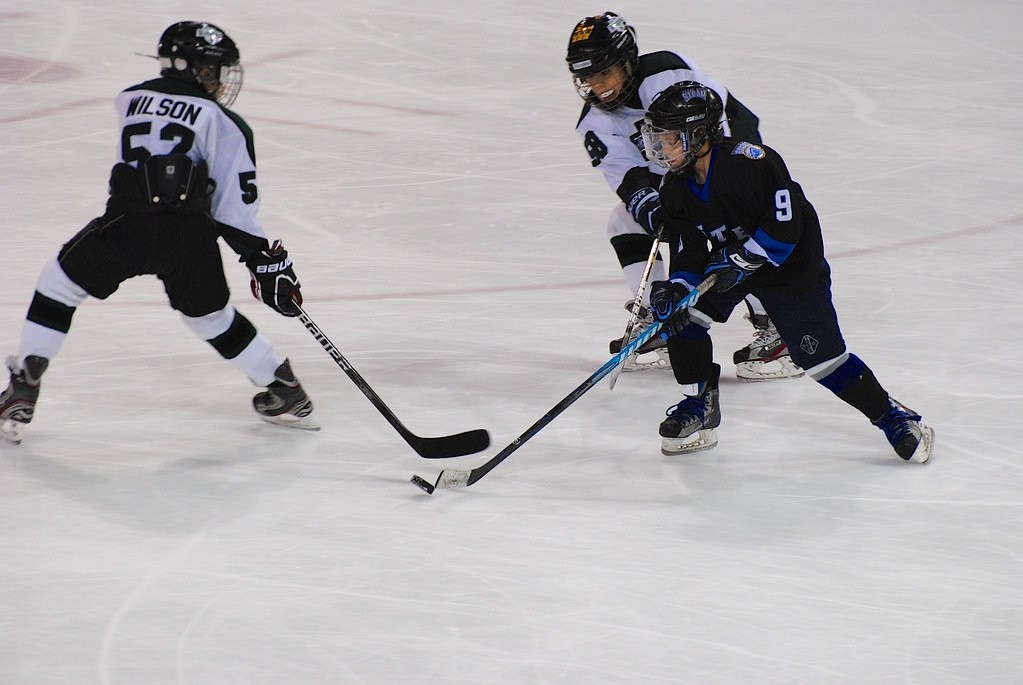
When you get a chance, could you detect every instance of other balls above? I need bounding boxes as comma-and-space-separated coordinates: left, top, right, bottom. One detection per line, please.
410, 475, 434, 496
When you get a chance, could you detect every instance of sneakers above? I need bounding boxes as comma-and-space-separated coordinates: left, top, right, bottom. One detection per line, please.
0, 355, 49, 443
659, 363, 721, 456
253, 358, 318, 430
733, 314, 805, 382
609, 298, 672, 373
870, 396, 934, 465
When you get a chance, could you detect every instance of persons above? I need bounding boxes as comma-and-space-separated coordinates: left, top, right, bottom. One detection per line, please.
0, 20, 321, 445
640, 81, 936, 467
564, 11, 806, 382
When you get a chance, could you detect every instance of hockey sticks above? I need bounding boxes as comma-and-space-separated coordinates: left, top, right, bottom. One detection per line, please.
434, 274, 714, 492
292, 298, 491, 460
606, 230, 664, 391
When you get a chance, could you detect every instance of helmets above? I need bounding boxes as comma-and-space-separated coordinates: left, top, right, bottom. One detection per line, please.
639, 80, 724, 177
157, 20, 244, 109
566, 11, 638, 112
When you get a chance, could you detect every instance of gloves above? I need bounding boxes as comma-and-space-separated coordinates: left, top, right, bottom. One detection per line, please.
649, 280, 692, 341
239, 239, 302, 316
703, 239, 767, 293
625, 187, 673, 243
